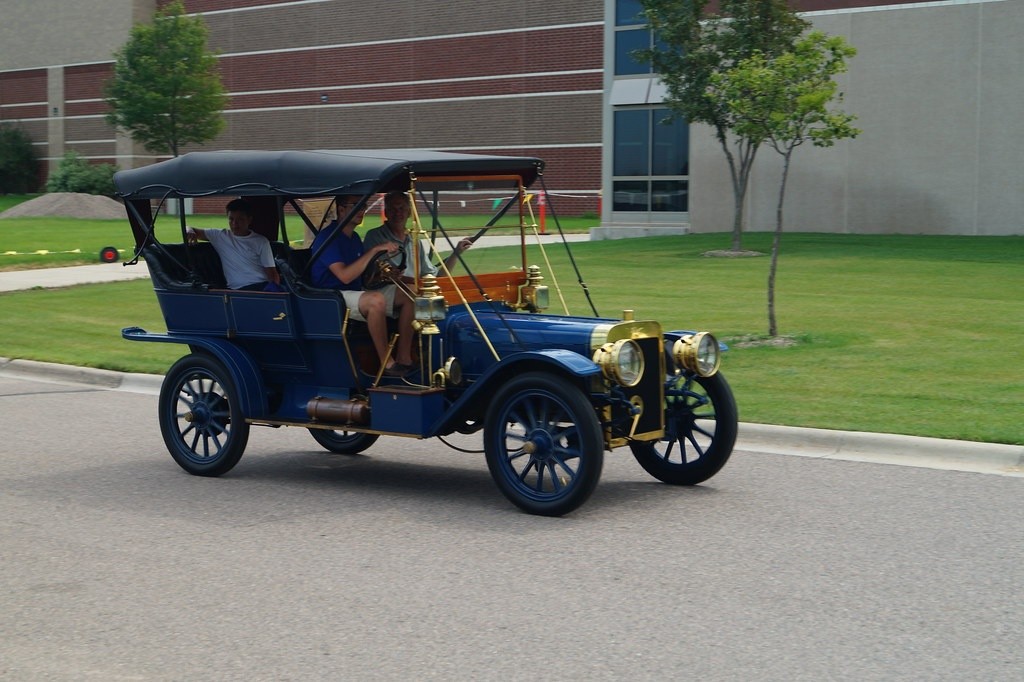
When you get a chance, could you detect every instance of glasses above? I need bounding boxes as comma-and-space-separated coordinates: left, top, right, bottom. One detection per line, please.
347, 202, 368, 211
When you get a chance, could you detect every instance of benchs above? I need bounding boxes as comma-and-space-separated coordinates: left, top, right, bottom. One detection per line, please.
142, 240, 294, 291
273, 247, 365, 335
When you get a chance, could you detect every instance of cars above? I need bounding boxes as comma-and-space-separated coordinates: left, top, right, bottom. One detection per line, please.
112, 146, 739, 519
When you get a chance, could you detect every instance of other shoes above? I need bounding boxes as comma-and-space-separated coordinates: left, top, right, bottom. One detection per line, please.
384, 362, 413, 375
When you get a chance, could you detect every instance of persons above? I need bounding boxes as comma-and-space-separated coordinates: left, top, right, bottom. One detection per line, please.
364, 192, 473, 283
186, 199, 280, 291
310, 195, 426, 376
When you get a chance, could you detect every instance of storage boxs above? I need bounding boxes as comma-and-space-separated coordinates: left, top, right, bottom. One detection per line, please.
367, 384, 446, 435
